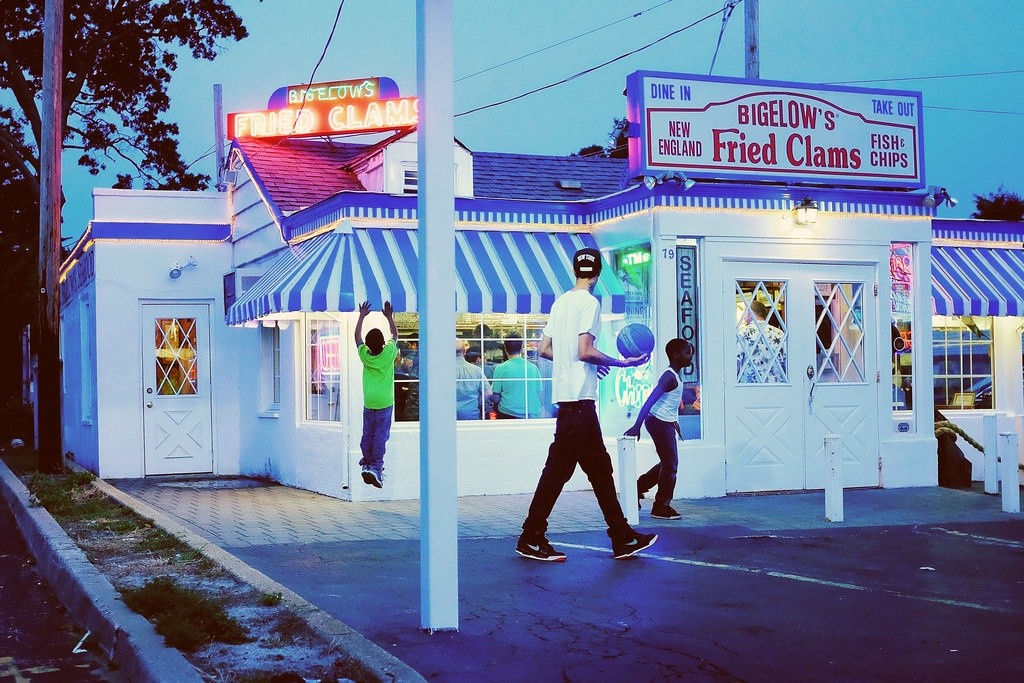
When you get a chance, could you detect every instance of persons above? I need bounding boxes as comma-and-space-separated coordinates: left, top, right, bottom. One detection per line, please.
623, 338, 693, 520
515, 249, 658, 562
735, 301, 786, 383
395, 323, 558, 420
355, 300, 398, 489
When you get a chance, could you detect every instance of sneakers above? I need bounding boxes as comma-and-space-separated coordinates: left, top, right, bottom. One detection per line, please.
614, 534, 658, 558
651, 506, 682, 519
515, 533, 566, 561
638, 495, 645, 511
361, 470, 383, 488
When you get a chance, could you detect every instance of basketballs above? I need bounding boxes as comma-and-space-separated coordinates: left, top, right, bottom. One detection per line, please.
617, 323, 655, 358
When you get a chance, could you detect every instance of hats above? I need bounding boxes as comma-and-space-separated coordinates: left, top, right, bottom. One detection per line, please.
573, 249, 602, 272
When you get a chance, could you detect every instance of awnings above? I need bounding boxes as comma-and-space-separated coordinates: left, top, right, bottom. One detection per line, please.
891, 244, 1024, 317
224, 228, 625, 329
